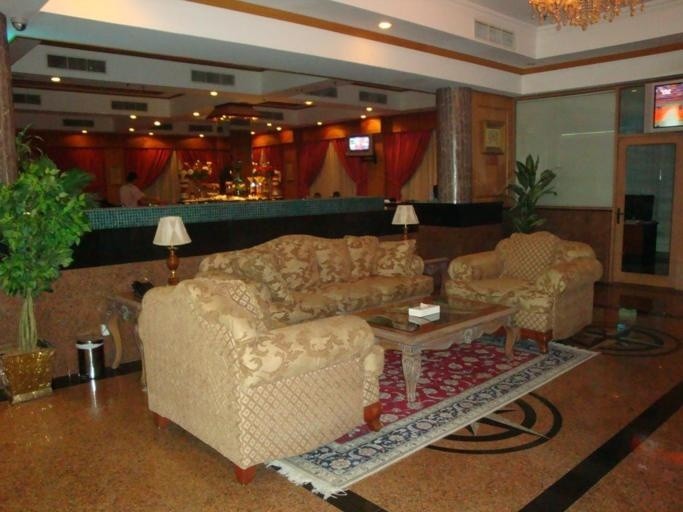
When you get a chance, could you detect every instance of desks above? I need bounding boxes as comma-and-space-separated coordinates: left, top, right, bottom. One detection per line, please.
420, 251, 448, 287
98, 285, 148, 392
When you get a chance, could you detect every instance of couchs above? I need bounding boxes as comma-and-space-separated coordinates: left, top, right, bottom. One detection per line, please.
207, 227, 436, 326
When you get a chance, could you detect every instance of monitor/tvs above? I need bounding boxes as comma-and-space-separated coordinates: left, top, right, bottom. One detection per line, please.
642, 78, 683, 134
347, 135, 373, 155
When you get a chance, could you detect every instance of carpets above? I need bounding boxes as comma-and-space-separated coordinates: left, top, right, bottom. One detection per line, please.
263, 331, 601, 501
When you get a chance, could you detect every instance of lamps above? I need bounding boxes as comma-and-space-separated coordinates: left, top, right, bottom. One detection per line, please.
150, 214, 196, 286
389, 197, 424, 242
527, 1, 648, 35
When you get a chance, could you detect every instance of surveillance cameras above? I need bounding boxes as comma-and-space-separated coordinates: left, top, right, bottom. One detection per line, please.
11, 17, 28, 31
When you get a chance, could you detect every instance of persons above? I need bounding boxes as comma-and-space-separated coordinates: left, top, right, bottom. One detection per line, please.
313, 192, 322, 199
431, 184, 438, 204
654, 83, 682, 127
332, 191, 340, 198
355, 139, 364, 149
118, 171, 146, 207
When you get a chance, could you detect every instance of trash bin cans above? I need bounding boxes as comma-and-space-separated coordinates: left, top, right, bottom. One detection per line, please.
75, 337, 105, 379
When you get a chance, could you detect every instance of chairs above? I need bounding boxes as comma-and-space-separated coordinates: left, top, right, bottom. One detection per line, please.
440, 229, 604, 358
136, 270, 387, 486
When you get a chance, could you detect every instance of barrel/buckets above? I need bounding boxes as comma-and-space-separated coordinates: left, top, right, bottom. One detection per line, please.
618, 307, 638, 327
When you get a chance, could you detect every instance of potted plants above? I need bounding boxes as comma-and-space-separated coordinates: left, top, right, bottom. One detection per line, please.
0, 152, 108, 407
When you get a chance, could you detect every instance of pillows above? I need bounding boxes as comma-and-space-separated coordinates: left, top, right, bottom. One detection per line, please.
498, 232, 558, 284
